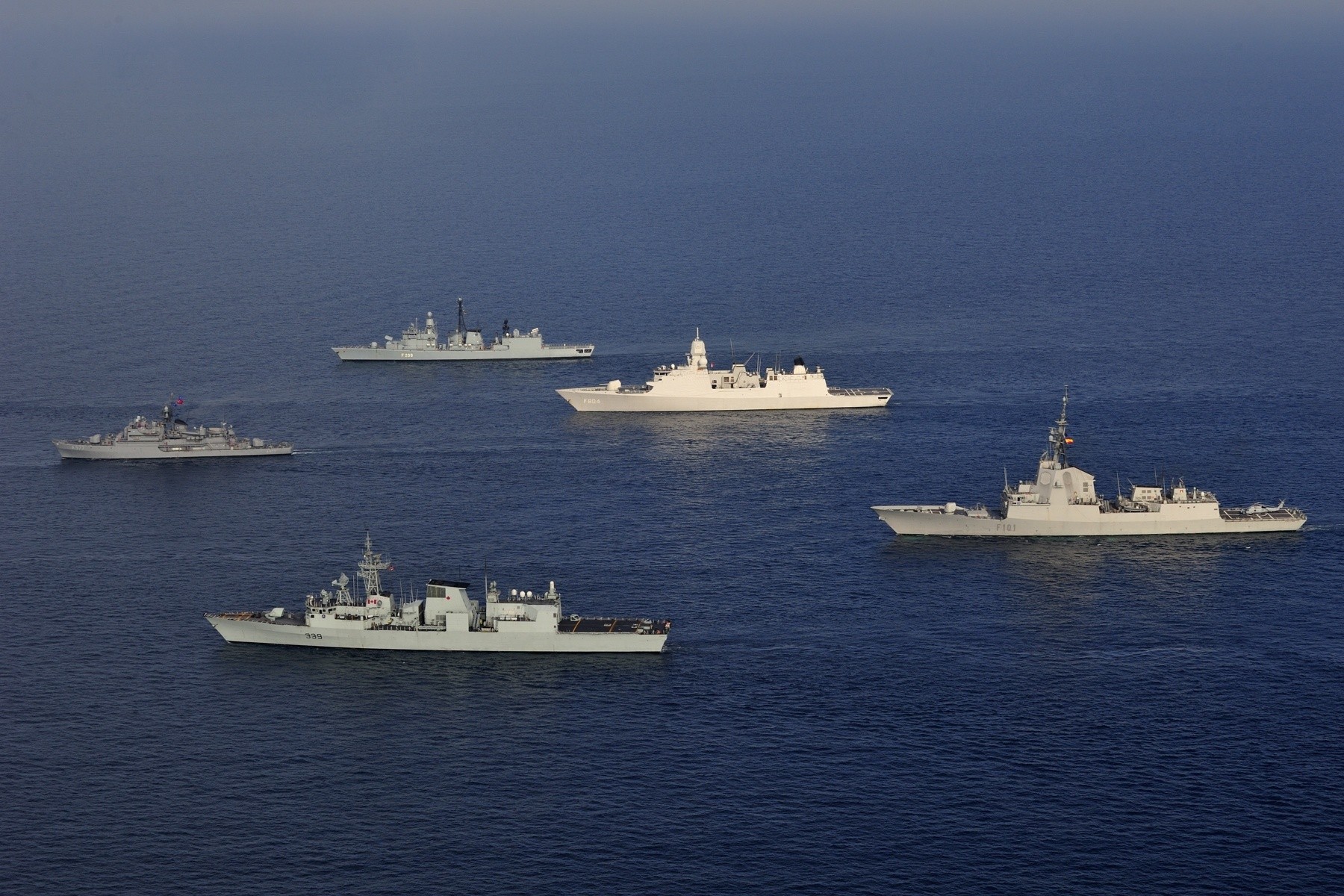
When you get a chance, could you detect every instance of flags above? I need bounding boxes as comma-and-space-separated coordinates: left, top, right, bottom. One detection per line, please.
173, 401, 177, 406
389, 566, 394, 571
176, 396, 184, 405
1063, 430, 1074, 448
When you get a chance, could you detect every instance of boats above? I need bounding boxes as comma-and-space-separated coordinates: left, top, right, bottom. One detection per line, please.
549, 323, 897, 414
199, 529, 674, 655
48, 391, 294, 460
865, 382, 1309, 538
327, 299, 600, 360
